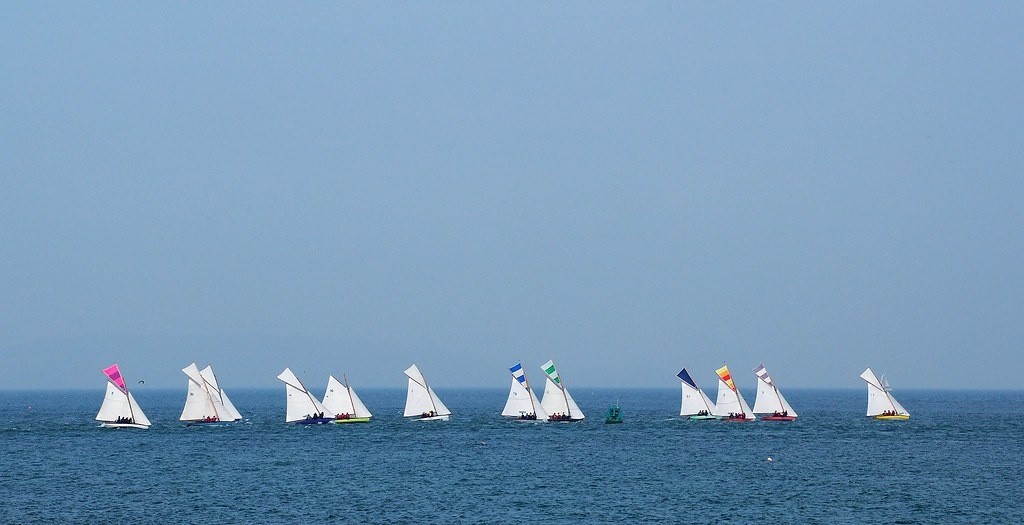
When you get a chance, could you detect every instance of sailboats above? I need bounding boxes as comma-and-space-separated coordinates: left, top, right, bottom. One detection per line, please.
752, 364, 798, 422
403, 363, 452, 422
323, 372, 373, 423
860, 368, 911, 421
540, 360, 586, 421
181, 363, 243, 426
94, 363, 152, 430
675, 367, 723, 421
276, 367, 336, 425
501, 363, 550, 421
714, 366, 757, 422
604, 400, 623, 424
